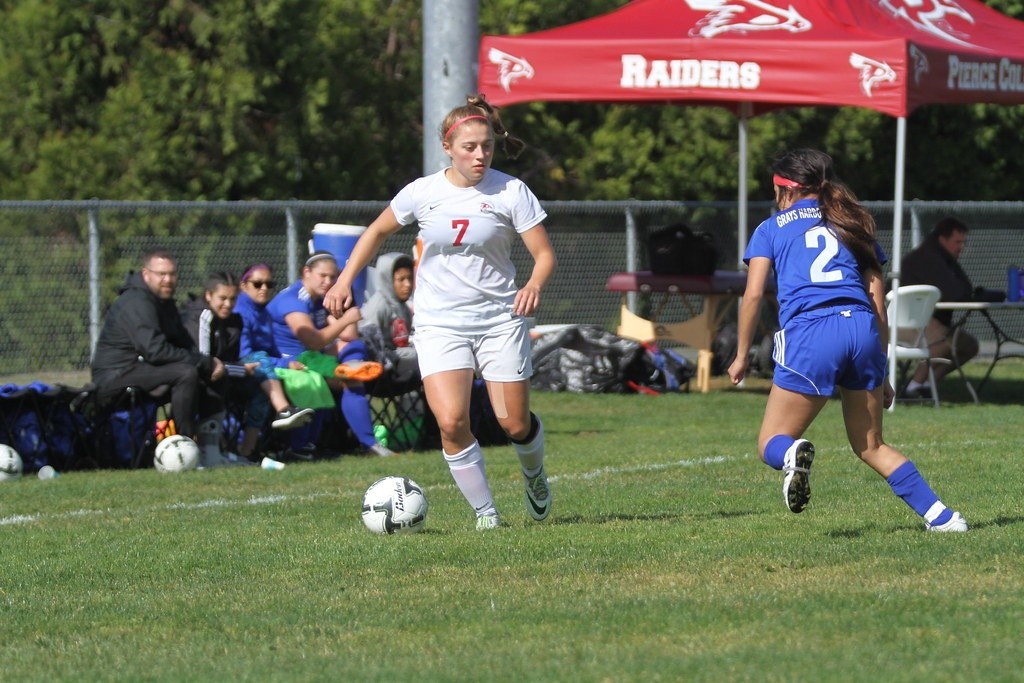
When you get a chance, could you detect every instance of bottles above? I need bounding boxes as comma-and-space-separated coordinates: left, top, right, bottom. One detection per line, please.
1008, 264, 1022, 302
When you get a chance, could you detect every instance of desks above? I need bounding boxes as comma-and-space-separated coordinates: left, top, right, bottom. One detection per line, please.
605, 271, 780, 391
929, 301, 1024, 402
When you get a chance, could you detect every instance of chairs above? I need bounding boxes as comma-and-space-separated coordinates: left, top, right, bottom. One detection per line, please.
877, 284, 942, 412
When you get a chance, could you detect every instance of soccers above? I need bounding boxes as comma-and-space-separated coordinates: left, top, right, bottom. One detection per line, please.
154, 434, 201, 476
0, 443, 24, 481
361, 475, 428, 535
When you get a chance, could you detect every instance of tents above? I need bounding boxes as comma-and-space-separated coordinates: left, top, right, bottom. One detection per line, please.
477, 0, 1024, 415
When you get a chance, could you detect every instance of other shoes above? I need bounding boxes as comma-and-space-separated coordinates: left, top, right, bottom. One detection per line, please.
355, 443, 399, 458
902, 385, 933, 400
335, 358, 383, 382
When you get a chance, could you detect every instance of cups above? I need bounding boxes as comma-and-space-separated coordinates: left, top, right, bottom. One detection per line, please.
260, 458, 284, 470
38, 465, 58, 479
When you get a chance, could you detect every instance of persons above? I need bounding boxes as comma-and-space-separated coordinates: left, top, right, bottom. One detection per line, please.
728, 148, 969, 533
884, 218, 980, 405
321, 93, 559, 533
100, 248, 444, 462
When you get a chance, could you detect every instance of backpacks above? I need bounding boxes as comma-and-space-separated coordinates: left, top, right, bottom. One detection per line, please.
618, 340, 698, 394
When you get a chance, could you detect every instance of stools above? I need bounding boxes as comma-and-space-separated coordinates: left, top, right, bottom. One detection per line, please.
0, 380, 433, 472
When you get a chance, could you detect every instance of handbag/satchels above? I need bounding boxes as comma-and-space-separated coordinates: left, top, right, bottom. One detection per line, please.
642, 224, 719, 277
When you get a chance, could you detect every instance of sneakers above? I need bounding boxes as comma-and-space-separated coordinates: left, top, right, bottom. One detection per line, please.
782, 439, 815, 513
924, 511, 969, 533
223, 406, 315, 474
476, 506, 501, 533
521, 465, 551, 522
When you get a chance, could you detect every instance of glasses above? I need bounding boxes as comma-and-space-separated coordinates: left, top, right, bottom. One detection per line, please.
244, 278, 277, 291
145, 267, 179, 280
